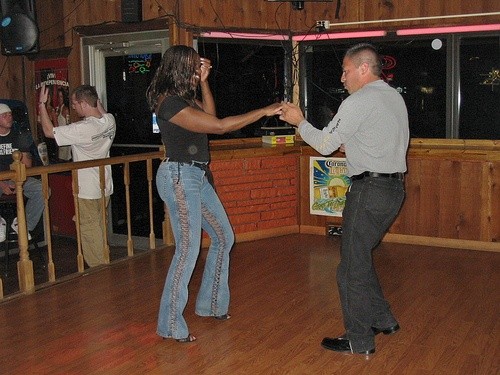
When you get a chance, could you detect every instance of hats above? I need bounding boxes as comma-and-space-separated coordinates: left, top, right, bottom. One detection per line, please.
0, 103, 12, 114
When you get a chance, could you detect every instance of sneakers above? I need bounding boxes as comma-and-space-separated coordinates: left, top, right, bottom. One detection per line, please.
11, 217, 32, 241
0, 218, 7, 242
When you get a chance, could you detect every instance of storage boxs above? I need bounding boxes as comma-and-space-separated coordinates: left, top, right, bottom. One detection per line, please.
261, 127, 295, 145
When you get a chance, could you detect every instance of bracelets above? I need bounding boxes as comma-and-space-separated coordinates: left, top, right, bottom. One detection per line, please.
38, 101, 45, 105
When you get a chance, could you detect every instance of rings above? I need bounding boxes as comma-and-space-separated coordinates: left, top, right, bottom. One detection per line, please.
209, 65, 213, 69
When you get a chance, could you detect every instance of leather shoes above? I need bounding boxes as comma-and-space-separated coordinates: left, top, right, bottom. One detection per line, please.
372, 325, 400, 335
321, 337, 375, 355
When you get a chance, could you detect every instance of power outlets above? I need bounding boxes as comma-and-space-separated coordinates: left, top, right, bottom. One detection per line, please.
315, 20, 330, 31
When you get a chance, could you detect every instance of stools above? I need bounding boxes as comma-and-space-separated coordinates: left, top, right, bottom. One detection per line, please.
0, 194, 47, 277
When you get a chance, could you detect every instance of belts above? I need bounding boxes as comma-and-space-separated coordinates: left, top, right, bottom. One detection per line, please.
350, 170, 405, 181
163, 158, 209, 172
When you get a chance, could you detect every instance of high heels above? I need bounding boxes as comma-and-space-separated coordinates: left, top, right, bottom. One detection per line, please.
199, 313, 232, 321
162, 334, 197, 343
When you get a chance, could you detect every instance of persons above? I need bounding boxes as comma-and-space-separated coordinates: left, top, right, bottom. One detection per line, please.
0, 103, 52, 246
145, 43, 291, 344
38, 82, 117, 268
39, 86, 74, 163
278, 43, 410, 355
